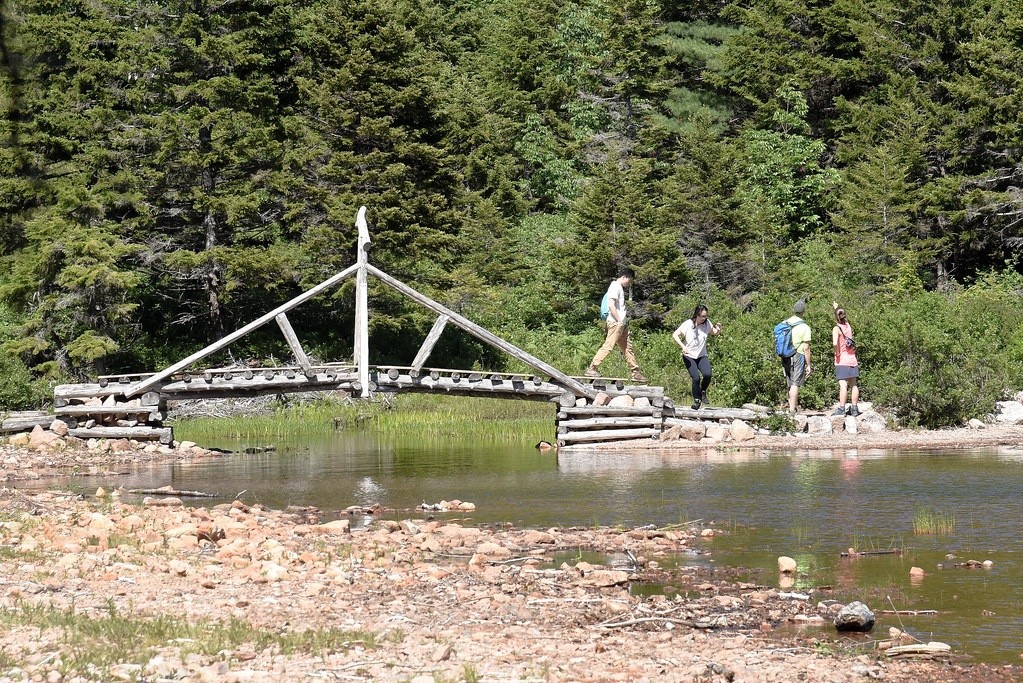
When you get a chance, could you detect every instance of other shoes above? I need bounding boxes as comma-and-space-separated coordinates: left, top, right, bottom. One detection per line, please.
835, 407, 845, 417
846, 406, 858, 417
789, 408, 804, 416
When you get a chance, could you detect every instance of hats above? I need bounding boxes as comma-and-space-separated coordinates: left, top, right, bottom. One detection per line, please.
794, 300, 805, 313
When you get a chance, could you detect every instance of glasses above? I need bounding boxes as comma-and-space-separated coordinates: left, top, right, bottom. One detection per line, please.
698, 314, 708, 318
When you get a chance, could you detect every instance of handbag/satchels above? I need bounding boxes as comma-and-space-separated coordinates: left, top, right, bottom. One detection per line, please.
844, 336, 857, 353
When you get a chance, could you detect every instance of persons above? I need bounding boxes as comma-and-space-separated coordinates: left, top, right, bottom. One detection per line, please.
780, 301, 812, 415
585, 267, 648, 383
671, 306, 722, 409
834, 300, 862, 416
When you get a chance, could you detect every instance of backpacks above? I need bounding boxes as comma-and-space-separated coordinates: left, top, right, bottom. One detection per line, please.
773, 321, 806, 357
600, 281, 623, 321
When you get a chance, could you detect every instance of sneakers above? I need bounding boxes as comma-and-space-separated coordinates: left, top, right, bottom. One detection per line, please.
691, 399, 701, 410
584, 368, 602, 378
700, 390, 709, 405
631, 373, 648, 382
